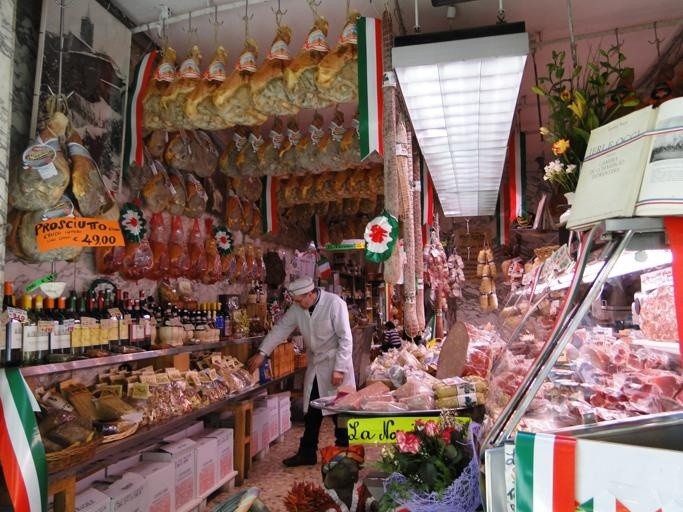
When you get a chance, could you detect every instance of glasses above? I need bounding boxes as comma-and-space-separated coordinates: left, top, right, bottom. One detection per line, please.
289, 296, 306, 303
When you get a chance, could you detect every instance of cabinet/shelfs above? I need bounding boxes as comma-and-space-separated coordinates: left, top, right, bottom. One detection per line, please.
0, 333, 295, 512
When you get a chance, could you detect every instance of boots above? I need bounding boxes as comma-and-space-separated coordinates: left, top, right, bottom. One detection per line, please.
282, 436, 318, 466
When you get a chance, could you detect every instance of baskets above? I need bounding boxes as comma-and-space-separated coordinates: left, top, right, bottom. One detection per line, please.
44, 432, 103, 473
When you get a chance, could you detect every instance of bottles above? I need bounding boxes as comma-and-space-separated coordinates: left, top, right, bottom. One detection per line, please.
250, 280, 260, 304
0, 282, 232, 367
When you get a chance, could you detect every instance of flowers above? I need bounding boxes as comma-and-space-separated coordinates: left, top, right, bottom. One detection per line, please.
532, 41, 640, 191
369, 418, 468, 496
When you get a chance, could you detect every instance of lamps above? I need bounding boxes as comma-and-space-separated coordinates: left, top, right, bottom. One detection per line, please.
392, 0, 529, 218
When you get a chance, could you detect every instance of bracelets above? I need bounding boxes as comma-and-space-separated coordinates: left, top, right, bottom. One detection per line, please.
257, 350, 267, 359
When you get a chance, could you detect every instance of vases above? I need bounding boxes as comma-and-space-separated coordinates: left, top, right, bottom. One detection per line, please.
560, 192, 577, 225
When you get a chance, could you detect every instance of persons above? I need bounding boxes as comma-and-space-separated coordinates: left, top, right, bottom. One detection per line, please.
381, 320, 401, 353
246, 275, 357, 467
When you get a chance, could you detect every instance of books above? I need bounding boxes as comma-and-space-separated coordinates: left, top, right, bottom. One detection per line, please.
565, 95, 683, 232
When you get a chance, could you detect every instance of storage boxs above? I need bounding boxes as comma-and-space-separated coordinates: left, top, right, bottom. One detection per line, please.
47, 420, 234, 512
219, 389, 292, 456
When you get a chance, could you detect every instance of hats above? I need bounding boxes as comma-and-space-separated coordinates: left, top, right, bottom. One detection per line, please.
286, 276, 315, 297
